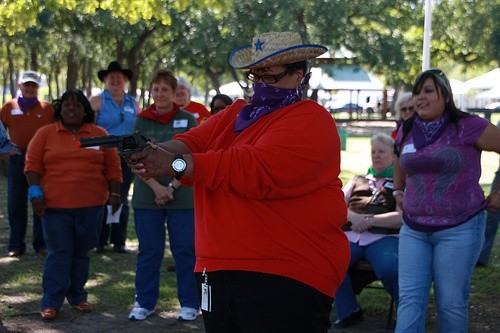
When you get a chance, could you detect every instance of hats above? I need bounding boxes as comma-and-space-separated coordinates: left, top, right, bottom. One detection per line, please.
98, 61, 133, 82
21, 72, 41, 86
229, 32, 328, 69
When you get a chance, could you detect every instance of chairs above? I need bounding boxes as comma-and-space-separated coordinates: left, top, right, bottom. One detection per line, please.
353, 258, 395, 329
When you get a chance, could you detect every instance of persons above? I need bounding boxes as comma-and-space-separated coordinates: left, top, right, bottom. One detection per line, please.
332, 133, 399, 328
0, 120, 19, 161
24, 88, 123, 320
362, 96, 375, 119
128, 70, 202, 322
174, 74, 211, 126
392, 95, 416, 139
88, 61, 142, 255
377, 102, 382, 111
210, 93, 233, 116
0, 70, 58, 258
129, 30, 352, 333
395, 69, 500, 333
475, 168, 500, 268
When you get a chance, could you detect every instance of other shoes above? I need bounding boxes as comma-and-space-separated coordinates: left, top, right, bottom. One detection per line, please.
93, 247, 104, 254
8, 250, 24, 257
37, 248, 49, 255
333, 306, 363, 328
113, 245, 128, 254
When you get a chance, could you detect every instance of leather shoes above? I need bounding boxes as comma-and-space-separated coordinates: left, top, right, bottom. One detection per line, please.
40, 308, 56, 322
74, 301, 93, 312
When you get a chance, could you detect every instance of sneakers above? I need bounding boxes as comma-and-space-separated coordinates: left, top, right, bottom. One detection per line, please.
176, 307, 202, 320
127, 308, 156, 320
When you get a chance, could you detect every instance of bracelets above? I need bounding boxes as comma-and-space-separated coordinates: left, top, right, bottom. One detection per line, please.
392, 189, 403, 196
28, 184, 43, 203
110, 192, 121, 197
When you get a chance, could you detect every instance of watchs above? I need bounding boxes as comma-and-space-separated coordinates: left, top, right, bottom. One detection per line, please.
168, 182, 176, 191
365, 216, 374, 223
170, 153, 187, 179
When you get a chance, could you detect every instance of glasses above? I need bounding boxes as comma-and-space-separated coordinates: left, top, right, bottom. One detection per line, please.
213, 105, 225, 111
246, 69, 290, 83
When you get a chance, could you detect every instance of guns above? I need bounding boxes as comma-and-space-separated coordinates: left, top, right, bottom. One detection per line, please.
74, 132, 153, 165
